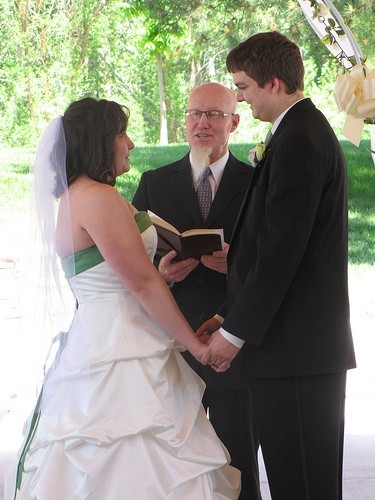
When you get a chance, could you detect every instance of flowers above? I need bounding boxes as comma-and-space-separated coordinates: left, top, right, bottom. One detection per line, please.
248, 140, 267, 167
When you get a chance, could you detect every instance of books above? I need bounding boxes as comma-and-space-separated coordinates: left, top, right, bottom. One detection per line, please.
147, 210, 224, 261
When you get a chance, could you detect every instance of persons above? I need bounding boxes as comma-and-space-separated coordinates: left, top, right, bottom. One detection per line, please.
195, 31, 357, 500
3, 93, 241, 500
131, 83, 255, 408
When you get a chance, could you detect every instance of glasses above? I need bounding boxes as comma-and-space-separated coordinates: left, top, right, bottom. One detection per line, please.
185, 109, 233, 120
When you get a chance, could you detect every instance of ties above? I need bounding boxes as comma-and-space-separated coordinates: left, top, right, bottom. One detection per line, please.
264, 128, 273, 145
197, 167, 213, 223
